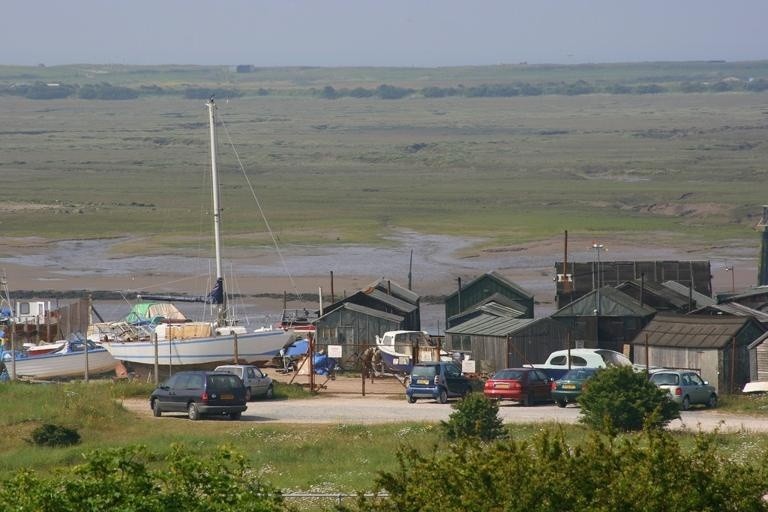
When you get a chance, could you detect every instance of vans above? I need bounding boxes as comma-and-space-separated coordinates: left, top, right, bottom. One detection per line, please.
649, 371, 717, 411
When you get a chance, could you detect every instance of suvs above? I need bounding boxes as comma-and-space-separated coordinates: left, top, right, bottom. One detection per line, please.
406, 360, 473, 404
150, 371, 247, 420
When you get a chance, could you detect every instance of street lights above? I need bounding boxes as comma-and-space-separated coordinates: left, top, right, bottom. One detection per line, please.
593, 244, 603, 314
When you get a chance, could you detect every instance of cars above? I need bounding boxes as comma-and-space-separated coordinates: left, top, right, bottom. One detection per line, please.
214, 365, 274, 401
523, 348, 656, 369
485, 368, 554, 406
551, 368, 598, 408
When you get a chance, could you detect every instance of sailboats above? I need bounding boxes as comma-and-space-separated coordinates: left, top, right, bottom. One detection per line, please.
88, 100, 295, 368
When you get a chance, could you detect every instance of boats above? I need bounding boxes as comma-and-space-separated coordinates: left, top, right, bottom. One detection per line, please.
3, 339, 121, 384
375, 330, 453, 373
